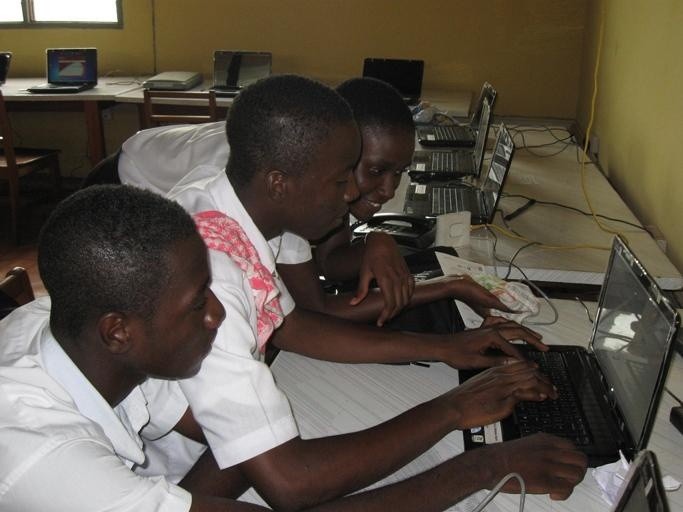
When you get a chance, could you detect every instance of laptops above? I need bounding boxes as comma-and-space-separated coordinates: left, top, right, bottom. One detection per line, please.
26, 48, 97, 94
403, 122, 516, 224
361, 58, 425, 107
0, 51, 12, 85
407, 96, 491, 178
416, 81, 497, 147
458, 234, 679, 469
205, 50, 272, 96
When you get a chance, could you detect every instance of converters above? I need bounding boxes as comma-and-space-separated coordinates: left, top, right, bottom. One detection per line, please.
670, 407, 682, 432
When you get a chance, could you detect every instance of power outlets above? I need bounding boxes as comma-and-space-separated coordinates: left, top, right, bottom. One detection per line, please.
586, 132, 598, 153
645, 223, 666, 254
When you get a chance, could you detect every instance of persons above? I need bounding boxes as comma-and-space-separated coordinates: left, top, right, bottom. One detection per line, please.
156, 74, 560, 508
0, 185, 591, 512
77, 76, 524, 326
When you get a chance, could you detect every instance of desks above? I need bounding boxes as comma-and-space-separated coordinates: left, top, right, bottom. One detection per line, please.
352, 129, 683, 304
117, 69, 472, 119
186, 285, 683, 512
1, 78, 152, 172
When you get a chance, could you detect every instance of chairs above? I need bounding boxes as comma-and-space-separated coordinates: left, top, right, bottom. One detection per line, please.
143, 89, 216, 126
0, 267, 35, 316
0, 91, 61, 245
